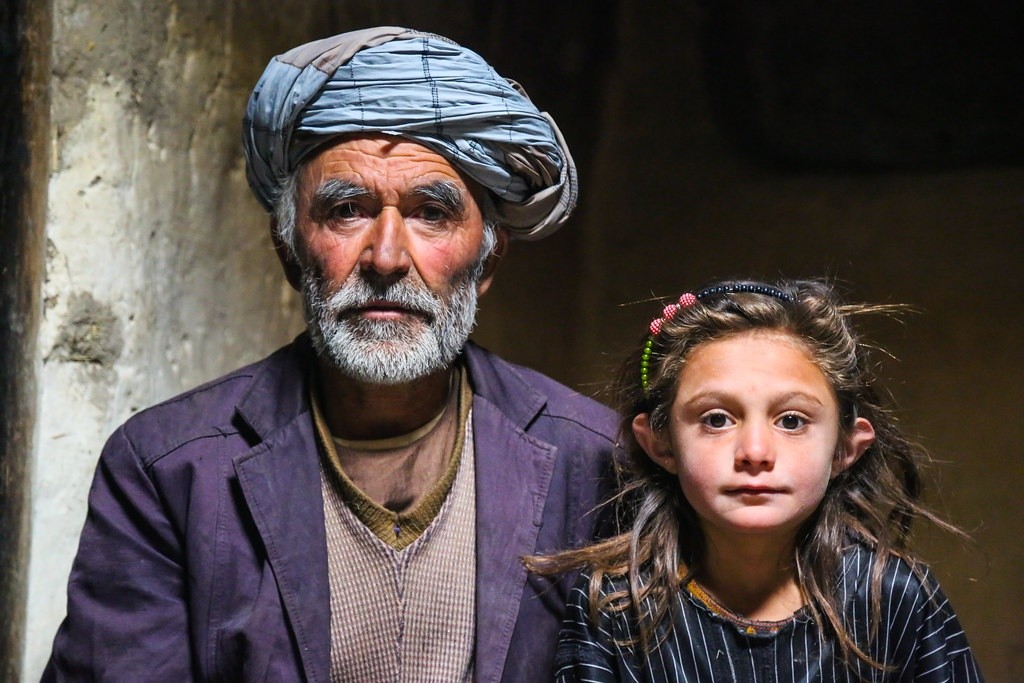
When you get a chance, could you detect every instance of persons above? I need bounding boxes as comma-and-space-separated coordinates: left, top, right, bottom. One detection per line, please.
38, 24, 625, 683
516, 278, 986, 683
